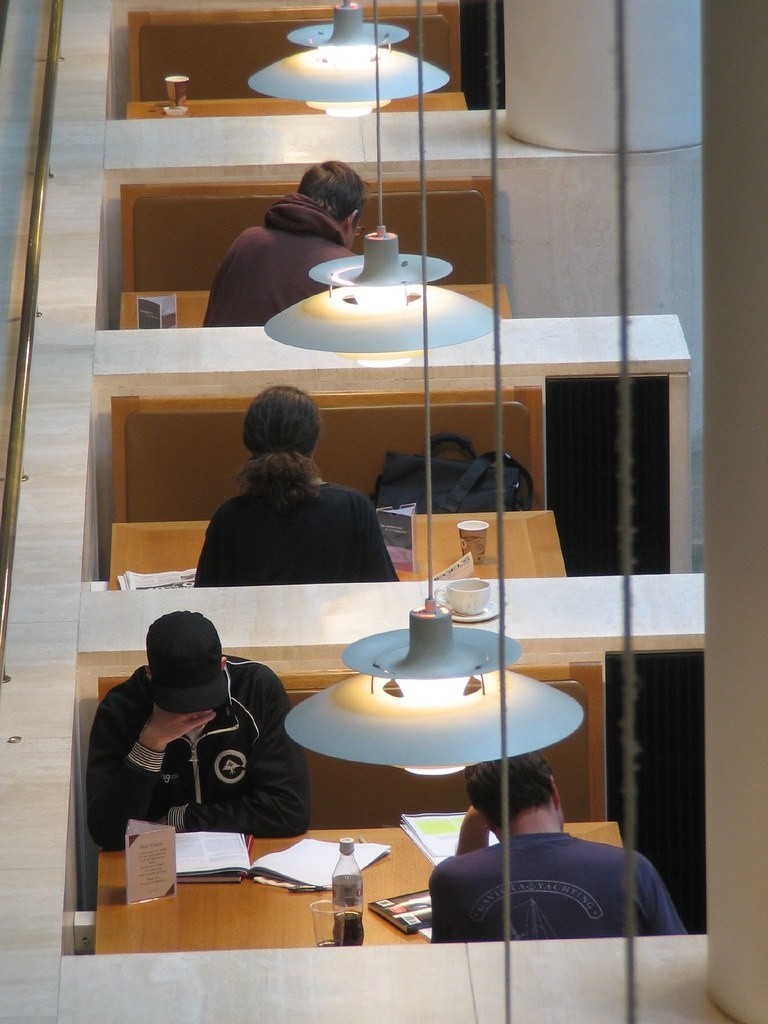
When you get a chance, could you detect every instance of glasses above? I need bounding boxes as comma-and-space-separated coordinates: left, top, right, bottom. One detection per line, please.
354, 217, 365, 235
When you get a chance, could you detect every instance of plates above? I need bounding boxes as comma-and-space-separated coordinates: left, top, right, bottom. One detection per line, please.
440, 602, 500, 622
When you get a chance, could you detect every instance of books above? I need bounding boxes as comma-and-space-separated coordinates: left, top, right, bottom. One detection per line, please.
368, 889, 432, 941
175, 835, 255, 883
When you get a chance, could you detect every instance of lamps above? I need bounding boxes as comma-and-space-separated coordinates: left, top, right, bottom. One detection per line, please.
283, 0, 586, 774
263, 2, 503, 367
247, 1, 450, 119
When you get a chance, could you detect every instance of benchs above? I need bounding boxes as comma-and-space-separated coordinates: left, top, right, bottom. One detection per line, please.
113, 383, 547, 510
119, 177, 500, 288
99, 662, 608, 830
130, 0, 465, 94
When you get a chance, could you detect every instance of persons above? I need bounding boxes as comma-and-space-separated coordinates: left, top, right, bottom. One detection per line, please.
429, 752, 689, 944
85, 610, 311, 850
201, 160, 366, 329
193, 386, 400, 588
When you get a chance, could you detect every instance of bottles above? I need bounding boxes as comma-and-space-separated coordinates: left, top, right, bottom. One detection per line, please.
332, 838, 364, 946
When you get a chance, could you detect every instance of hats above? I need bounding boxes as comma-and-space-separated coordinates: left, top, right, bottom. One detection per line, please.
146, 611, 227, 714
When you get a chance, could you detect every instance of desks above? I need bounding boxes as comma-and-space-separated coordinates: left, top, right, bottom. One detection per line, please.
118, 284, 511, 330
108, 510, 566, 590
127, 92, 469, 120
95, 822, 621, 956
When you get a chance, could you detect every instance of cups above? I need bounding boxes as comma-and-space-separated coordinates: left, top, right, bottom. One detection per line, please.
434, 579, 491, 616
457, 520, 489, 564
165, 76, 189, 107
309, 899, 347, 947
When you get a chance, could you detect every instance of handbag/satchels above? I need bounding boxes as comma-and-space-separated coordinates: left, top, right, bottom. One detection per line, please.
374, 431, 533, 515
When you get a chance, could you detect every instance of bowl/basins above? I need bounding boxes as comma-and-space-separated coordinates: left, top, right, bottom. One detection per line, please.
164, 106, 189, 116
